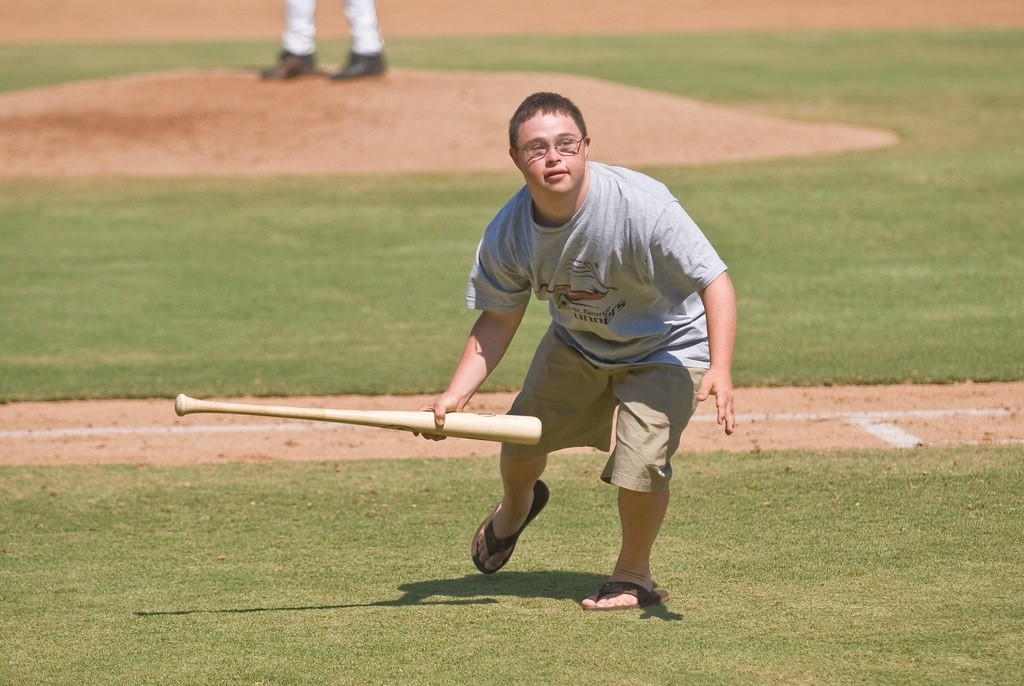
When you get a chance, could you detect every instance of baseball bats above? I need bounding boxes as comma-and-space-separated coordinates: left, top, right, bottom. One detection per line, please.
174, 393, 544, 446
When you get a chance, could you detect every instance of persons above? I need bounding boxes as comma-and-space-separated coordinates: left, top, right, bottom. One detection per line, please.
258, 0, 388, 83
413, 91, 737, 609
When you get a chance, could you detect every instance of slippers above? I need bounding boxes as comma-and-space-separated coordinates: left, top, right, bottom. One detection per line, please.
471, 479, 550, 574
582, 581, 669, 610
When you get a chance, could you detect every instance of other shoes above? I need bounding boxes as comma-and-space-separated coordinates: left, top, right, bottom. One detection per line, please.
260, 51, 314, 80
331, 51, 384, 81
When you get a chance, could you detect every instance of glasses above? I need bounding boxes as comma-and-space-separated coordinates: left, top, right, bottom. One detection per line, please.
513, 136, 583, 158
